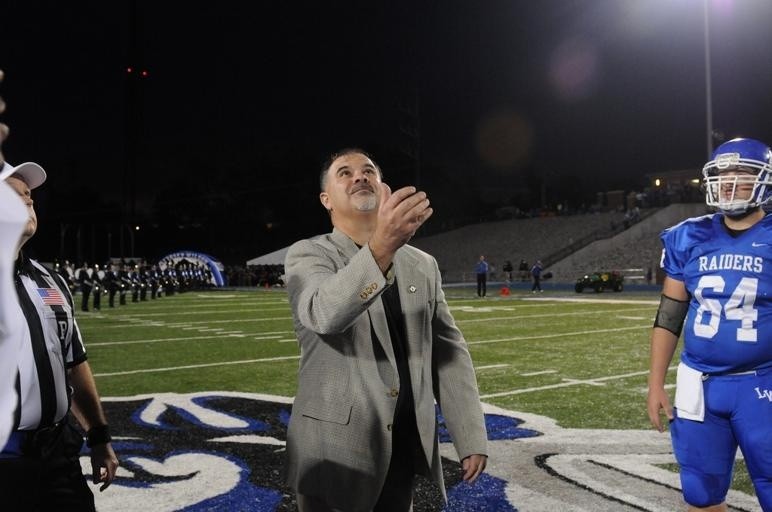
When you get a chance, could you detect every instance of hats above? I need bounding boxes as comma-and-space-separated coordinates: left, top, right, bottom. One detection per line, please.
1, 162, 46, 189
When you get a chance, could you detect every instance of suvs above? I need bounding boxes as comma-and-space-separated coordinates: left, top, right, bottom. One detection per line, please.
575, 270, 624, 293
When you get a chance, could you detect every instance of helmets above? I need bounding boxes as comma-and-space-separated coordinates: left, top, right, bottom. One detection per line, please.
702, 138, 771, 219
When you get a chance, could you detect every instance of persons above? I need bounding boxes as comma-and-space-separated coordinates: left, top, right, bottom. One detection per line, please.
85, 421, 112, 448
635, 184, 705, 211
529, 259, 544, 294
511, 194, 596, 219
45, 255, 286, 314
518, 259, 530, 282
607, 201, 643, 239
503, 260, 513, 282
1, 67, 34, 507
641, 134, 772, 512
279, 142, 491, 510
0, 155, 122, 511
646, 267, 652, 286
474, 255, 489, 300
488, 262, 500, 283
589, 269, 624, 284
